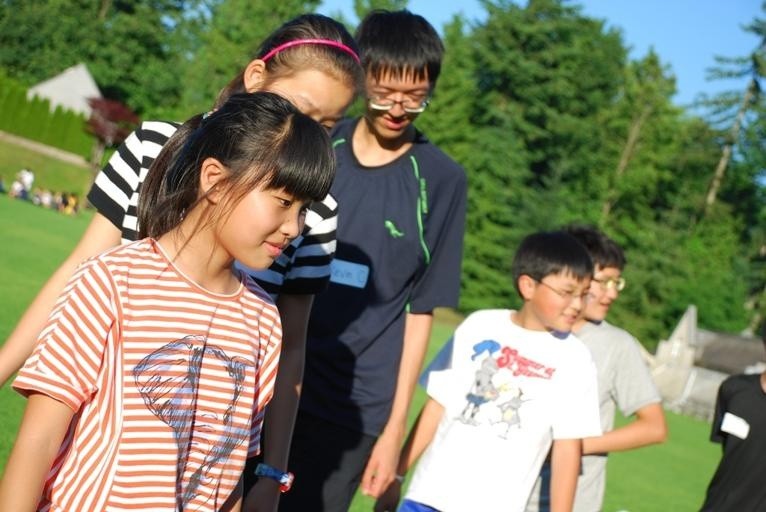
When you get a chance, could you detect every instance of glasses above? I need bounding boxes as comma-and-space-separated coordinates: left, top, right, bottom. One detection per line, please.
536, 278, 590, 298
365, 94, 430, 113
592, 276, 627, 291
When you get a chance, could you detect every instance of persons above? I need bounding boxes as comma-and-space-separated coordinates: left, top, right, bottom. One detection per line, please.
373, 227, 665, 511
2, 92, 335, 510
278, 10, 470, 511
10, 167, 79, 216
699, 320, 766, 512
1, 11, 366, 510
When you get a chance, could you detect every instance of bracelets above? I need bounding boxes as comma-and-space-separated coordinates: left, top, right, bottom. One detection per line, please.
255, 463, 295, 493
